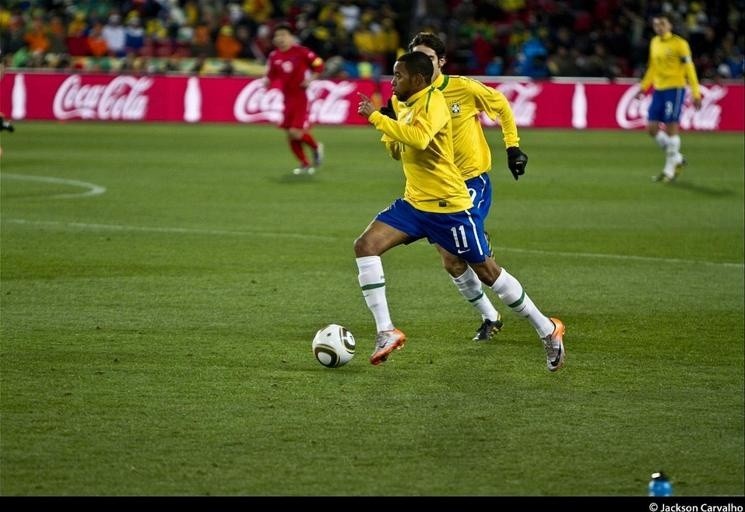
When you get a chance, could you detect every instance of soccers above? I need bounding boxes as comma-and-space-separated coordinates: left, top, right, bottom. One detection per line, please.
312, 324, 355, 368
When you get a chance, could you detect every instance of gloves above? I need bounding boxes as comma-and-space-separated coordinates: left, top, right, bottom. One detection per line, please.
505, 147, 528, 180
380, 99, 397, 121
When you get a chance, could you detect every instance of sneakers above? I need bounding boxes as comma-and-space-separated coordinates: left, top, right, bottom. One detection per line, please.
472, 313, 504, 343
539, 317, 566, 372
674, 154, 687, 178
314, 143, 323, 165
370, 328, 406, 365
655, 170, 674, 184
293, 167, 314, 176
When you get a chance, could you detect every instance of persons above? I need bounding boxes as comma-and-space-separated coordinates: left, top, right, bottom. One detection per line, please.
386, 32, 528, 341
637, 12, 704, 183
263, 25, 328, 176
1, 1, 743, 81
355, 51, 565, 372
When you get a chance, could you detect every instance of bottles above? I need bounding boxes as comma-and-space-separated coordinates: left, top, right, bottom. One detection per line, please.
572, 83, 589, 131
183, 75, 203, 124
9, 73, 27, 121
649, 469, 672, 496
